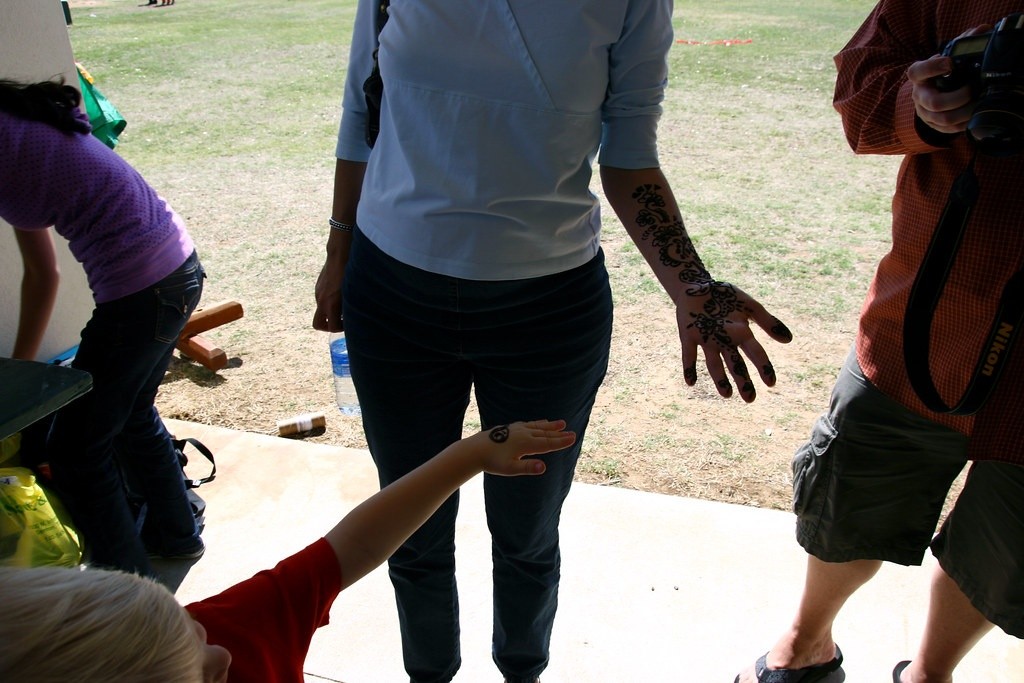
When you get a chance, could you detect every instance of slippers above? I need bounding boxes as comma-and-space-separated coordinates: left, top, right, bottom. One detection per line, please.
893, 660, 911, 683
734, 642, 844, 683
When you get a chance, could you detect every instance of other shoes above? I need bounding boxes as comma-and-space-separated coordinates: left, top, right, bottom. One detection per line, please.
143, 534, 206, 561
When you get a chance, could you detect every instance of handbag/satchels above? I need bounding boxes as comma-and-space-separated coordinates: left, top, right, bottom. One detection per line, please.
168, 433, 216, 528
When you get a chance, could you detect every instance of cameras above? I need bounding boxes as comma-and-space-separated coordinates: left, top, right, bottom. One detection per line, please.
935, 13, 1024, 156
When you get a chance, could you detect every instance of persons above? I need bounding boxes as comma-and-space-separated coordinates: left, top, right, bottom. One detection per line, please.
0, 79, 206, 577
1, 417, 578, 683
312, 1, 795, 683
746, 0, 1024, 683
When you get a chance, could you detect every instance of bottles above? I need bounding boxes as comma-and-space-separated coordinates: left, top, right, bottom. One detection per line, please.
330, 331, 362, 415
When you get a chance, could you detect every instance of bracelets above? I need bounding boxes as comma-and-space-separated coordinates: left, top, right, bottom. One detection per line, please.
329, 218, 353, 231
